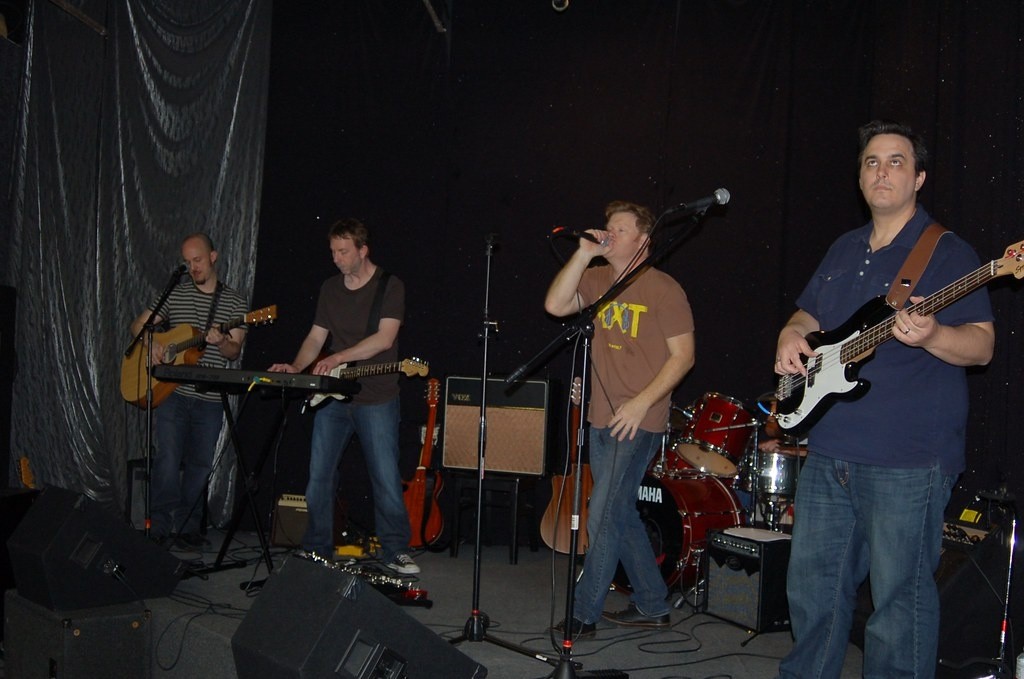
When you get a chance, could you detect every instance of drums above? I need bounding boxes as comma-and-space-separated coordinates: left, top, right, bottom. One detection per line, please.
731, 448, 804, 498
612, 451, 745, 598
673, 390, 756, 475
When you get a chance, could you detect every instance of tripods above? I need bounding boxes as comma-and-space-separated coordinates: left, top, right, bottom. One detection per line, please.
447, 229, 584, 670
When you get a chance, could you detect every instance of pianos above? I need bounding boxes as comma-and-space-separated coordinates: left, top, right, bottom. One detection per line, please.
147, 364, 364, 580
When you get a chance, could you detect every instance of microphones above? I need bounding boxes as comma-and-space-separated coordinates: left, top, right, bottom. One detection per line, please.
174, 262, 191, 274
562, 226, 610, 247
665, 188, 730, 215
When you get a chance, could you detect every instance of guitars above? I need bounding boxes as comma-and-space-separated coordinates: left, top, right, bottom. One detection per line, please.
541, 378, 596, 554
117, 302, 280, 411
300, 356, 431, 417
771, 237, 1019, 438
401, 376, 461, 553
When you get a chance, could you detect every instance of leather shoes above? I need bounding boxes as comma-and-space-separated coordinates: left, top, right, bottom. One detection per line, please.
544, 619, 597, 639
603, 604, 671, 629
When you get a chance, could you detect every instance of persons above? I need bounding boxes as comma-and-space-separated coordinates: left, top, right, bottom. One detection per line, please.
545, 202, 694, 639
267, 220, 421, 574
773, 124, 995, 679
130, 233, 249, 551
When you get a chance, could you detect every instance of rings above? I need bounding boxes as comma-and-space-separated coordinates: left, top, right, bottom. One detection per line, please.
904, 328, 910, 335
775, 358, 780, 363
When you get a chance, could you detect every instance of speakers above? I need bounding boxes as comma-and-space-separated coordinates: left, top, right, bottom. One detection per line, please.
703, 528, 792, 633
271, 492, 310, 546
8, 482, 189, 612
916, 516, 1024, 679
231, 554, 488, 679
443, 374, 560, 481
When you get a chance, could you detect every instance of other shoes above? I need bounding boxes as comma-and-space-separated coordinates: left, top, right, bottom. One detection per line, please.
151, 533, 168, 546
173, 531, 211, 551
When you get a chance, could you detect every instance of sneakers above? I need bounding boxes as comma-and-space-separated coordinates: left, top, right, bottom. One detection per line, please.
388, 553, 421, 575
